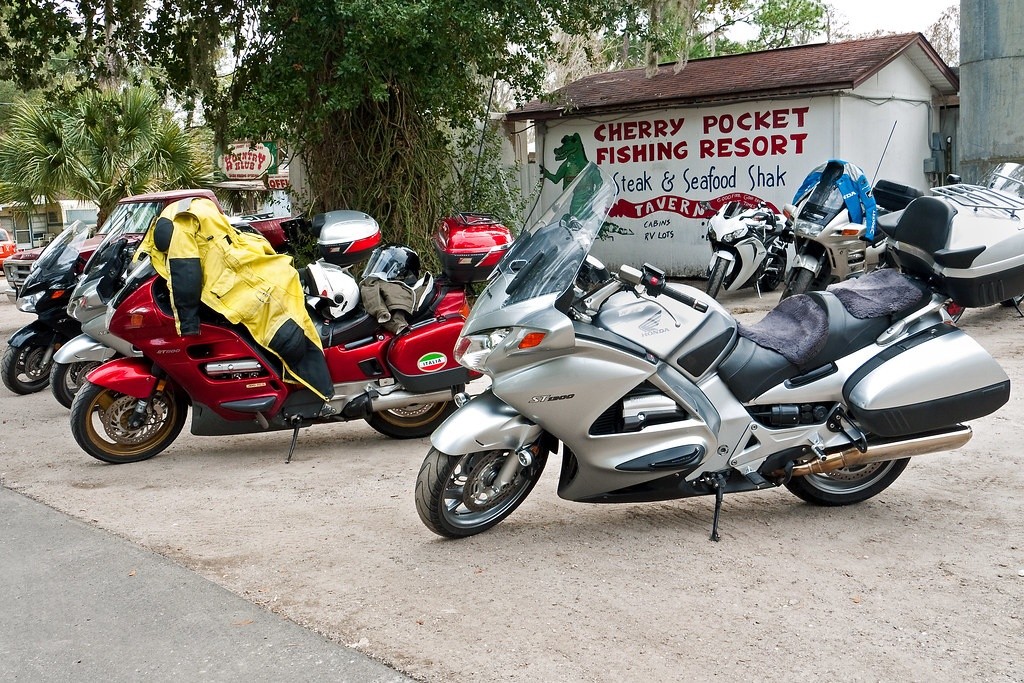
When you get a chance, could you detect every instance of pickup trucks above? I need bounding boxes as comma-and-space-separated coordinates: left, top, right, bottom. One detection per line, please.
3, 190, 304, 303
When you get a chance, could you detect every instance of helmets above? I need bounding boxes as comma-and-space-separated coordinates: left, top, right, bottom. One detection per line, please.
362, 243, 421, 287
302, 256, 360, 320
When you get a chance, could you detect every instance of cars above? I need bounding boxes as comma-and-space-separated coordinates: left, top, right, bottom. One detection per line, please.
0, 229, 16, 271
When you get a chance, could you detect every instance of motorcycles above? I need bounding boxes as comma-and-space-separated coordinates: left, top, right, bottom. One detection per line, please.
1, 193, 516, 464
413, 161, 1024, 541
